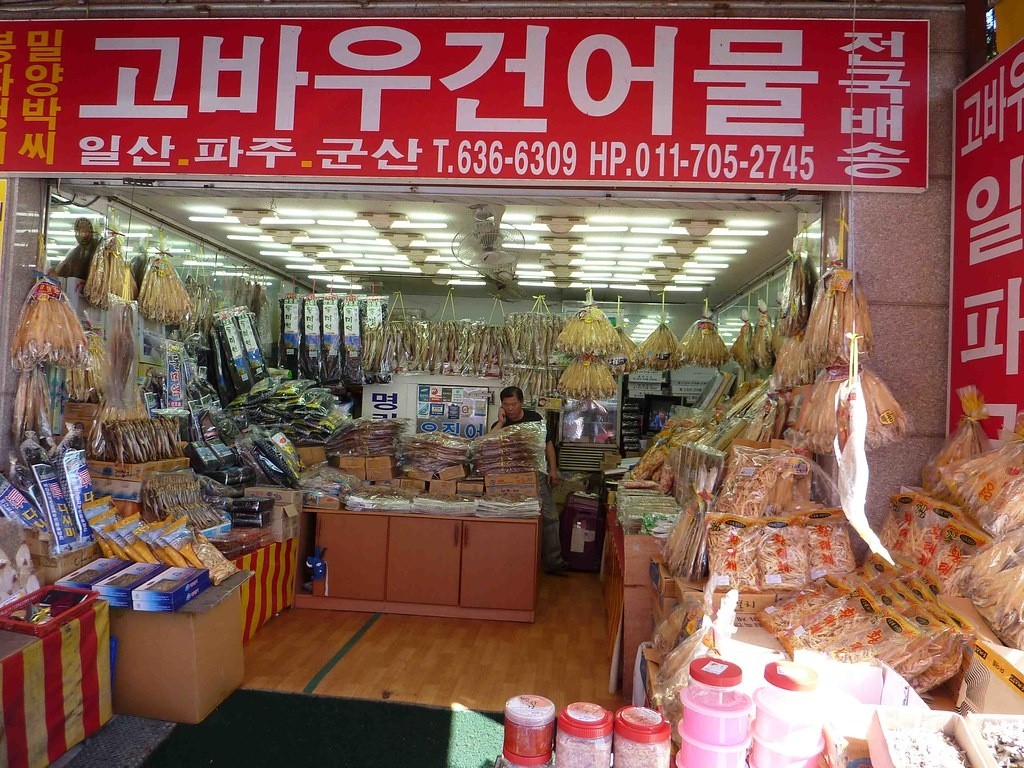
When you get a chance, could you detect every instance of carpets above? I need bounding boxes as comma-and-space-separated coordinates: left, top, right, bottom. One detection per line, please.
135, 688, 508, 768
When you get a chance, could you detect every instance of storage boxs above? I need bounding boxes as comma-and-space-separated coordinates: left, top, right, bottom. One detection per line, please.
622, 364, 719, 452
293, 445, 537, 500
61, 402, 100, 438
641, 552, 799, 743
244, 486, 303, 542
107, 568, 255, 724
599, 451, 622, 471
53, 556, 211, 614
84, 457, 194, 502
936, 592, 1024, 717
23, 529, 99, 587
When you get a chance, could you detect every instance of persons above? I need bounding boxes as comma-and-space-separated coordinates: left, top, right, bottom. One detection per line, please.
489, 386, 571, 576
654, 407, 670, 430
52, 218, 99, 280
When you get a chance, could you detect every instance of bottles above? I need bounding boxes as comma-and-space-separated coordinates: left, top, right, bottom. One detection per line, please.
760, 661, 821, 712
556, 702, 613, 768
503, 694, 555, 766
688, 659, 742, 707
613, 704, 671, 768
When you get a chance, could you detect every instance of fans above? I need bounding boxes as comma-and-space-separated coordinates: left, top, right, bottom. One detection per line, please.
450, 203, 527, 303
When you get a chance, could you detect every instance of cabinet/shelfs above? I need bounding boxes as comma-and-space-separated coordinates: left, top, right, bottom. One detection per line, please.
292, 509, 540, 624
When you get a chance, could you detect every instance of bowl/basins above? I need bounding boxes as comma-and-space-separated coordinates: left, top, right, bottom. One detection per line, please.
675, 687, 825, 768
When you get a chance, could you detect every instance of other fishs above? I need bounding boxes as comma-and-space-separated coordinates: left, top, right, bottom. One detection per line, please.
10, 234, 905, 553
